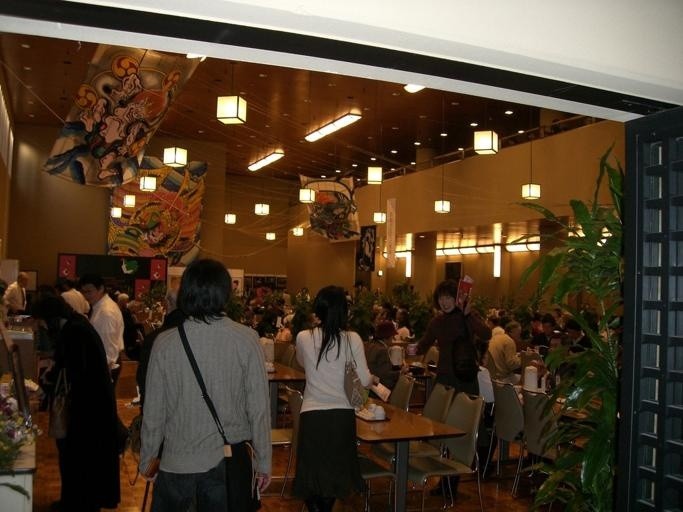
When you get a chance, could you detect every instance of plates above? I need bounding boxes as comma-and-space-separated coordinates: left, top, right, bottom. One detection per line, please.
355, 414, 391, 423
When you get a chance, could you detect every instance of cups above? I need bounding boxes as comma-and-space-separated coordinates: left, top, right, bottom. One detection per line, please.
368, 404, 376, 412
406, 343, 418, 356
375, 406, 385, 419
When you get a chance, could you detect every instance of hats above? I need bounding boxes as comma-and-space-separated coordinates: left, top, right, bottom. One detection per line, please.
375, 320, 399, 338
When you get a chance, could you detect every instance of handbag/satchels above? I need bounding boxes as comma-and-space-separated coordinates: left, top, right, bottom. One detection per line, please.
48, 366, 70, 439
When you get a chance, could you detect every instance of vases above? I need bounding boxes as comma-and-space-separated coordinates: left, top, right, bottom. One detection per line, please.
148, 310, 153, 319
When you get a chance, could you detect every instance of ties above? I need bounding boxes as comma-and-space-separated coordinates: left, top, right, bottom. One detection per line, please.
21, 288, 26, 308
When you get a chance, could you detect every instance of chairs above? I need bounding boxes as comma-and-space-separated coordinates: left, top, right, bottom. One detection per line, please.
512, 392, 586, 512
389, 374, 416, 411
264, 386, 303, 501
370, 383, 455, 512
483, 380, 542, 483
395, 392, 482, 512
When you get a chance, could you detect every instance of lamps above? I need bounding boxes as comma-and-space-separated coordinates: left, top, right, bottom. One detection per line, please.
265, 233, 275, 241
373, 212, 386, 224
224, 213, 236, 224
474, 131, 498, 155
382, 236, 535, 259
493, 223, 501, 279
123, 195, 135, 207
248, 152, 284, 173
163, 147, 187, 167
293, 228, 303, 237
217, 95, 245, 125
367, 167, 382, 185
299, 189, 314, 203
140, 176, 156, 192
111, 208, 122, 218
435, 201, 450, 213
522, 184, 541, 199
304, 112, 363, 143
254, 204, 269, 215
406, 232, 412, 278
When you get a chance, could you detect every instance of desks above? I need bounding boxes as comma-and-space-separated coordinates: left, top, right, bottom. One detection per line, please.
355, 398, 467, 512
266, 364, 305, 428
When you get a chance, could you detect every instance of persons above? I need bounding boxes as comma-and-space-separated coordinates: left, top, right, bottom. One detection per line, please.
2, 272, 31, 318
290, 301, 312, 343
532, 316, 566, 357
369, 303, 416, 343
140, 258, 273, 512
296, 284, 378, 511
565, 320, 592, 355
520, 316, 540, 342
116, 292, 131, 319
553, 346, 587, 383
489, 320, 522, 384
56, 273, 90, 318
264, 297, 285, 325
409, 279, 491, 401
78, 276, 124, 386
25, 287, 129, 512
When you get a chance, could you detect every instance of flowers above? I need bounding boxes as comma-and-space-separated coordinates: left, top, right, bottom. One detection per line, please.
0, 394, 43, 442
138, 283, 167, 309
